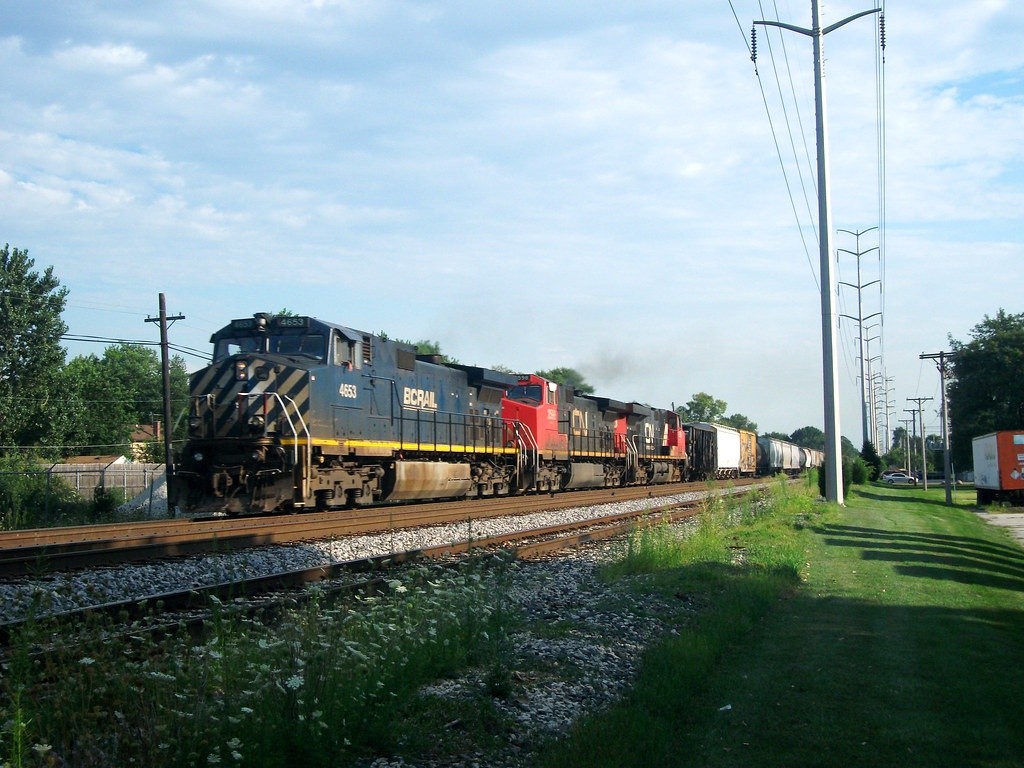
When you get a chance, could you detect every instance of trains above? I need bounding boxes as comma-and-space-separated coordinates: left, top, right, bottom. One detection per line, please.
169, 309, 826, 518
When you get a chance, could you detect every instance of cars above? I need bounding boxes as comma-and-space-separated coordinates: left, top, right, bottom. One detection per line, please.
883, 473, 919, 484
